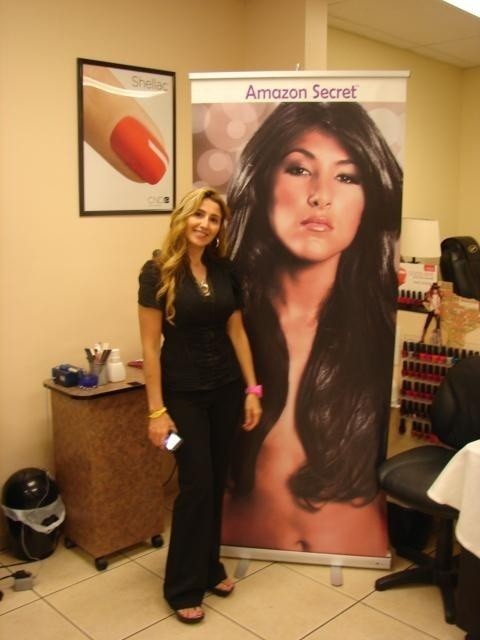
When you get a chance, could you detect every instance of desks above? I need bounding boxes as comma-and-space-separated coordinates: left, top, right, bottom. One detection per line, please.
42, 365, 166, 571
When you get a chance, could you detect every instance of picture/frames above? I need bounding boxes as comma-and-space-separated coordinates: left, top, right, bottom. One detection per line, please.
74, 56, 179, 219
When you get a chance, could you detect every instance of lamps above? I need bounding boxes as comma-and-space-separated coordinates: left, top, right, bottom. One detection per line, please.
398, 217, 442, 265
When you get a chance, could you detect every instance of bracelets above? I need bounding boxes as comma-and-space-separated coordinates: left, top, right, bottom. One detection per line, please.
148, 405, 168, 420
244, 385, 264, 400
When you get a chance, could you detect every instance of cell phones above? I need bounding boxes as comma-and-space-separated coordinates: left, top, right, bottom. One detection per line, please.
163, 433, 185, 452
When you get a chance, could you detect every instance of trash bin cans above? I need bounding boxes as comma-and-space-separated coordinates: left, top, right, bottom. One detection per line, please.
1, 468, 60, 562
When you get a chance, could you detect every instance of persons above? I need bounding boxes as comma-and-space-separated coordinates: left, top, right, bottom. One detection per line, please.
217, 101, 404, 559
417, 283, 442, 345
83, 64, 169, 185
135, 185, 265, 621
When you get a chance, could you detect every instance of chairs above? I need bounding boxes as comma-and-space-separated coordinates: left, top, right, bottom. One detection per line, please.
372, 352, 480, 623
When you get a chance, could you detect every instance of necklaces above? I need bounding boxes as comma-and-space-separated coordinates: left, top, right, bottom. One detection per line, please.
194, 268, 210, 296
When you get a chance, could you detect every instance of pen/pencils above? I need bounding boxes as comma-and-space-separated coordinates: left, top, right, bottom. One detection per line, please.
85, 348, 111, 376
